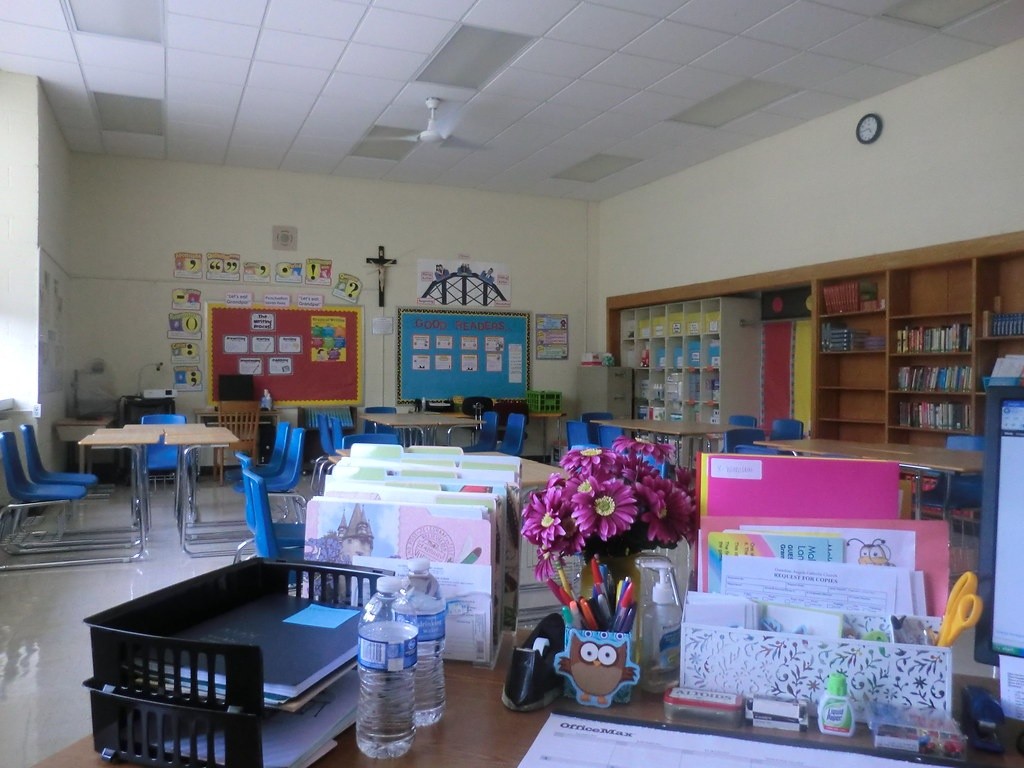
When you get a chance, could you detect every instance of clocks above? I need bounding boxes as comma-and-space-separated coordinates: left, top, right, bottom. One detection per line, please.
856, 114, 881, 143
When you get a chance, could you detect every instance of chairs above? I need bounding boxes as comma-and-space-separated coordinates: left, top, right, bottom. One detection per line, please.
214, 401, 260, 485
311, 396, 524, 496
0, 414, 305, 570
565, 412, 984, 520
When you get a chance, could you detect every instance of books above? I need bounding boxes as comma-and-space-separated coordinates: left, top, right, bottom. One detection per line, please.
822, 281, 885, 314
637, 311, 723, 471
982, 296, 1024, 386
120, 591, 376, 768
896, 322, 973, 430
821, 321, 886, 352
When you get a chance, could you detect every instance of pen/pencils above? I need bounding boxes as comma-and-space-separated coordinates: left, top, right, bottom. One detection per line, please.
546, 555, 638, 635
891, 615, 931, 645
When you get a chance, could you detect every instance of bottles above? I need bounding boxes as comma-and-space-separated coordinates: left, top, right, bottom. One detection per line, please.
638, 569, 682, 694
401, 558, 447, 727
355, 573, 417, 761
816, 672, 856, 738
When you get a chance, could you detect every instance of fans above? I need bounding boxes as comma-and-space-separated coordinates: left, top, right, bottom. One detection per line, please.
358, 99, 494, 157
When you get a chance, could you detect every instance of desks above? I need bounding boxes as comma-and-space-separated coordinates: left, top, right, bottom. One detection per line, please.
30, 627, 1024, 768
0, 423, 305, 570
590, 419, 983, 520
329, 414, 567, 625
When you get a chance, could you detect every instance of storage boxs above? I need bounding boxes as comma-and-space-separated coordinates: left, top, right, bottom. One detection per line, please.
525, 391, 562, 414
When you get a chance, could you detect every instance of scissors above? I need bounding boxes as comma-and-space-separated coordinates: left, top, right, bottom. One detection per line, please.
934, 571, 983, 647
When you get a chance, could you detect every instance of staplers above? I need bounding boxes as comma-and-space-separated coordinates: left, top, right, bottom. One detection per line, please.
962, 686, 1007, 750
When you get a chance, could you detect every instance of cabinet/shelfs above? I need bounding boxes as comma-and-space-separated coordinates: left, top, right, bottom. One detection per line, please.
620, 298, 764, 467
576, 366, 633, 420
813, 248, 1024, 448
